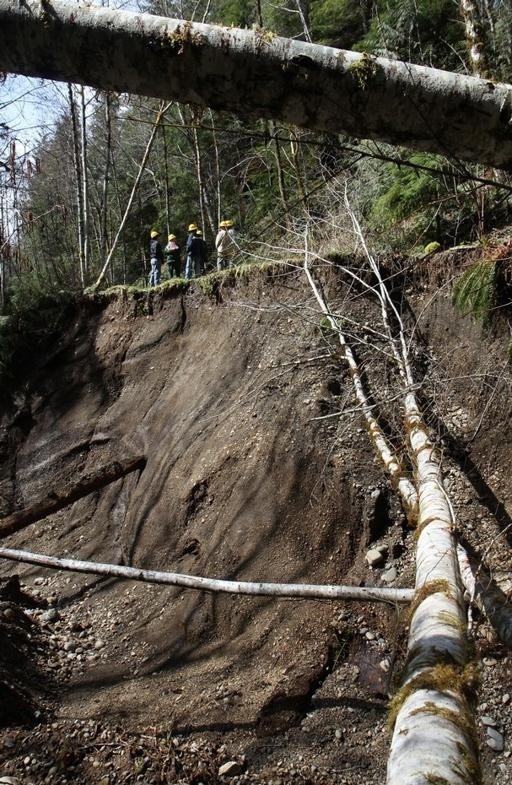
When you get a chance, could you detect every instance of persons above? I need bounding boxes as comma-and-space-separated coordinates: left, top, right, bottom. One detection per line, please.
196, 228, 207, 276
215, 221, 226, 272
149, 230, 161, 287
164, 233, 182, 279
227, 219, 237, 267
186, 223, 198, 280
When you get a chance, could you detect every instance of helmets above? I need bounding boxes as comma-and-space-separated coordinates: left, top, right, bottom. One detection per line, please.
167, 234, 176, 241
188, 223, 202, 236
220, 220, 234, 228
151, 231, 161, 239
